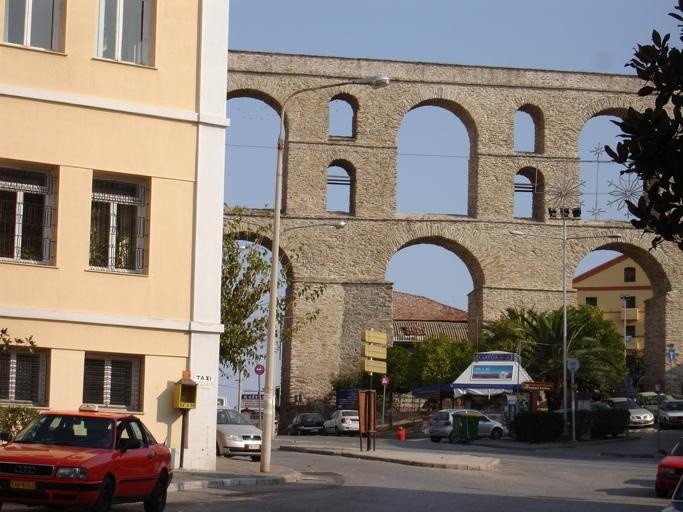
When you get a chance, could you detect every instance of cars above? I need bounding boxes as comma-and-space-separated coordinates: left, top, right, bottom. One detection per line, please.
217, 406, 262, 462
602, 391, 683, 427
0, 410, 173, 512
286, 412, 325, 436
655, 436, 683, 496
429, 408, 510, 443
322, 409, 360, 436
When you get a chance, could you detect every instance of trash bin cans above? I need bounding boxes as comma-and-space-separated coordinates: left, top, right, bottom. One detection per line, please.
452, 412, 481, 443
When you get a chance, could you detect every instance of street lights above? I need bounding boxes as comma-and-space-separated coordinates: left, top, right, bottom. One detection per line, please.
271, 220, 346, 439
261, 74, 390, 473
509, 230, 622, 442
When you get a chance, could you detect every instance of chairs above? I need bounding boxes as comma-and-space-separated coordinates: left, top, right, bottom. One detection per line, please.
54, 426, 141, 449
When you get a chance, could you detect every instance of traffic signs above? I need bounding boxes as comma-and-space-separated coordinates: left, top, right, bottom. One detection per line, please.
242, 395, 263, 400
